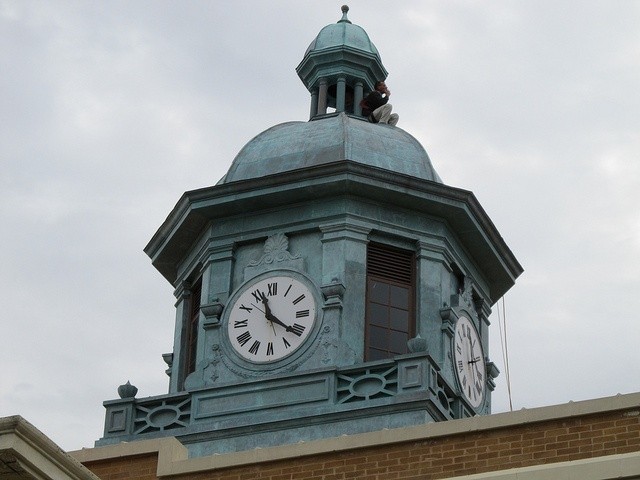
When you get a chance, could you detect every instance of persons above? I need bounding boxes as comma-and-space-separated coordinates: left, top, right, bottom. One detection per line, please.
362, 80, 399, 130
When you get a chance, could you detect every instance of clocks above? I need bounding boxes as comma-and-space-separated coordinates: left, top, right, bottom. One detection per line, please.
455, 316, 485, 408
227, 275, 318, 366
